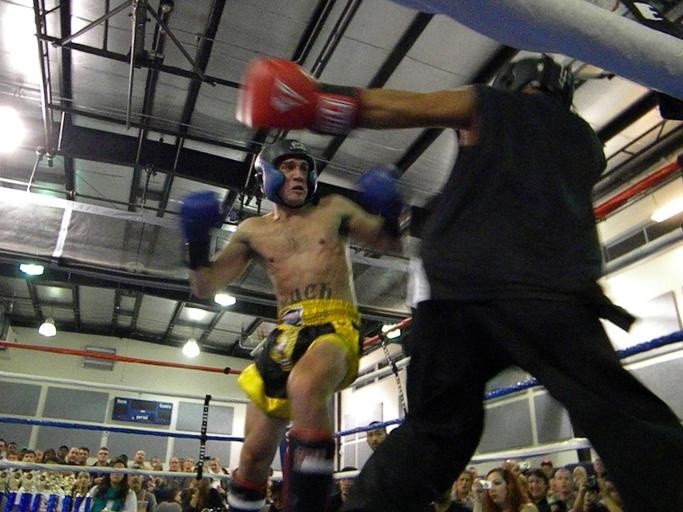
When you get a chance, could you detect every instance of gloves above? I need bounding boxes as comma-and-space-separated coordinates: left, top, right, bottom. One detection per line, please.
236, 58, 360, 136
352, 163, 412, 240
177, 191, 227, 271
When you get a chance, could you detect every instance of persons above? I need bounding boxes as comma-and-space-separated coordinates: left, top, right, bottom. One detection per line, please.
235, 51, 683, 512
180, 138, 403, 512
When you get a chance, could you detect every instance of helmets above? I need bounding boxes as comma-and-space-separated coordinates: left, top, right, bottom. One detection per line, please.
493, 53, 573, 110
254, 139, 318, 208
